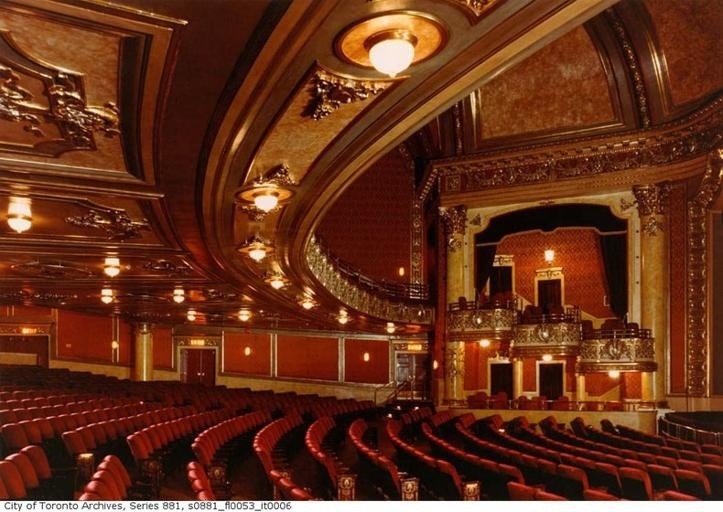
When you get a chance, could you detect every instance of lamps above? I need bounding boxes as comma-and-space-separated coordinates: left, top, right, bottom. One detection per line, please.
362, 27, 419, 79
250, 189, 281, 216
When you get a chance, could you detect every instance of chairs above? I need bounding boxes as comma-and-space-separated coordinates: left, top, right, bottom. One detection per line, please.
456, 291, 641, 337
